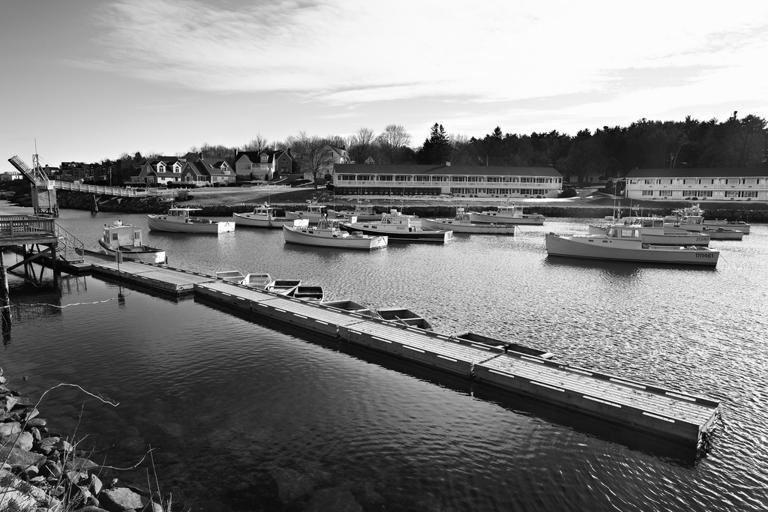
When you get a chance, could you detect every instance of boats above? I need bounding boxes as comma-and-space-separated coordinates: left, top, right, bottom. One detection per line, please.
283, 190, 389, 250
147, 198, 235, 235
98, 220, 165, 266
471, 191, 545, 226
236, 189, 294, 228
217, 271, 322, 305
422, 198, 515, 236
451, 332, 563, 365
589, 196, 750, 244
339, 184, 454, 245
323, 300, 380, 330
377, 309, 435, 337
547, 218, 720, 269
